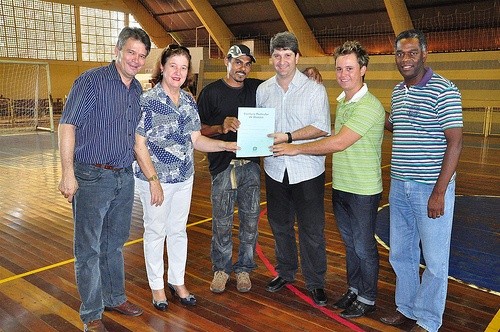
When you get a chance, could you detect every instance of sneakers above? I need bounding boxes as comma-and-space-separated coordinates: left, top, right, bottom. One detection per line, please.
210, 270, 230, 293
235, 272, 252, 292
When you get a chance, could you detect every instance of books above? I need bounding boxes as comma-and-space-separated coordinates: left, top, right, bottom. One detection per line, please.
236, 107, 275, 157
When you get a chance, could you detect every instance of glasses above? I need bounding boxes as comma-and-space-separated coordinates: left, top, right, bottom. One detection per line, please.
163, 43, 190, 56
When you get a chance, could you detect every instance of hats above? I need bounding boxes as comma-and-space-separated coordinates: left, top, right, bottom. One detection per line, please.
227, 43, 256, 63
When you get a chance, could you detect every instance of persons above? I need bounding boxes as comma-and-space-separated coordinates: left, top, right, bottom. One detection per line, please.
379, 29, 464, 332
131, 44, 241, 312
268, 39, 385, 318
196, 44, 323, 294
256, 31, 333, 306
57, 27, 151, 332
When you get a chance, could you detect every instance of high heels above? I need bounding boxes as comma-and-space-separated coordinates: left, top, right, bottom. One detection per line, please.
153, 296, 168, 311
167, 282, 197, 306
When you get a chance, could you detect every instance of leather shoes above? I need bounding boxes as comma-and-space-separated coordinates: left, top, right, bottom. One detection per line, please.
378, 311, 416, 326
338, 300, 376, 318
331, 289, 357, 310
264, 274, 295, 293
83, 319, 107, 332
311, 288, 328, 306
104, 299, 144, 317
409, 324, 428, 332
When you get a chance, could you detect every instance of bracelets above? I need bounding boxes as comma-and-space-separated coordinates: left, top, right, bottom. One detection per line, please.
148, 175, 159, 182
285, 132, 292, 143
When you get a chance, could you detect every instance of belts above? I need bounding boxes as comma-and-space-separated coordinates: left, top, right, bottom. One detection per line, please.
230, 159, 251, 166
95, 163, 113, 170
